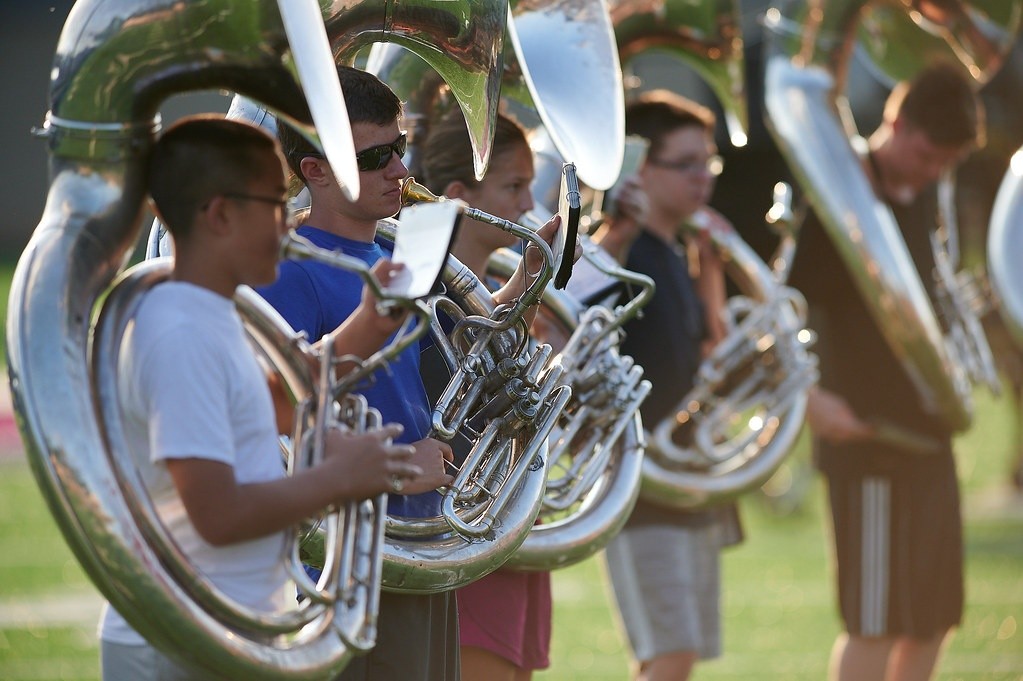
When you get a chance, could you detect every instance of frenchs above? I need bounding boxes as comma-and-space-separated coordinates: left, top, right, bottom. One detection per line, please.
2, 1, 1022, 681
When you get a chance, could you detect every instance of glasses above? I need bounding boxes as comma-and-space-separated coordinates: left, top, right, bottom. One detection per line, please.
290, 130, 408, 172
201, 193, 292, 225
647, 153, 725, 179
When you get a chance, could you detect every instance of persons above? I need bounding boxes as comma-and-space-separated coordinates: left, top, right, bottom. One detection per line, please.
558, 89, 744, 681
399, 109, 551, 681
787, 1, 1023, 680
256, 66, 583, 681
101, 115, 421, 680
